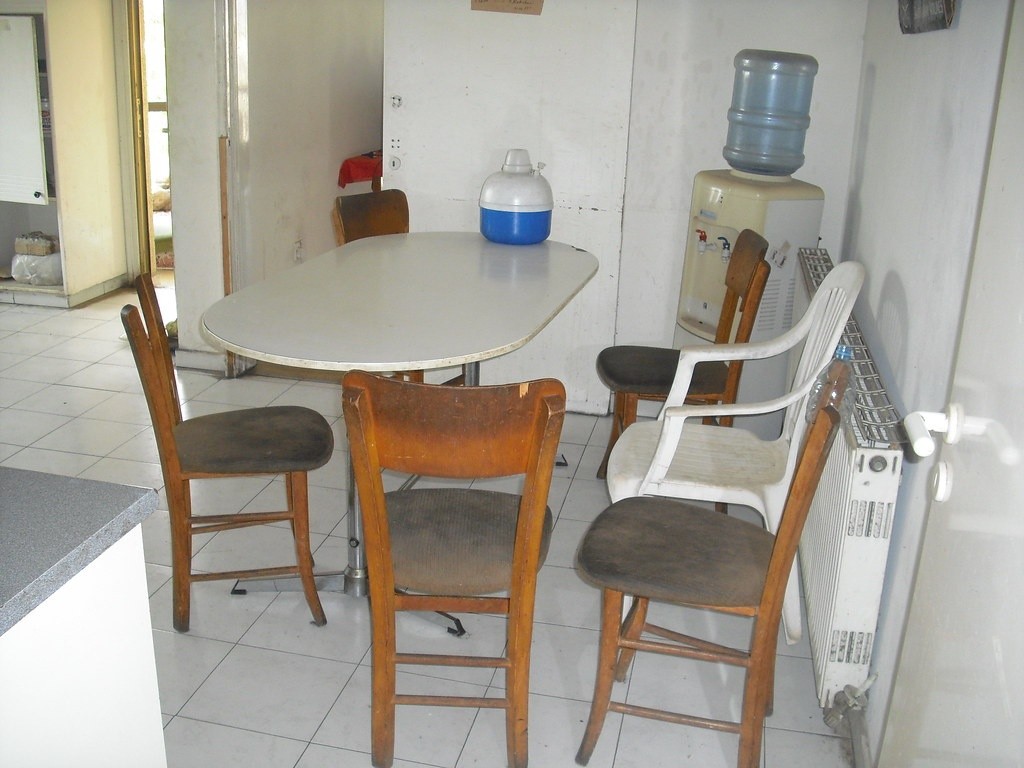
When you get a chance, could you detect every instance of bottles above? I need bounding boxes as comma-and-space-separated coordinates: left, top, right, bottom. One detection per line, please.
41, 97, 50, 130
723, 48, 819, 174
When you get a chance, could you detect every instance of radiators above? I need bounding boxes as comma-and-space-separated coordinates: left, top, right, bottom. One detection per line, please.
784, 247, 911, 709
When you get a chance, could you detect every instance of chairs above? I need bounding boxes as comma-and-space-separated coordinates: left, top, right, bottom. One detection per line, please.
333, 189, 466, 388
120, 273, 334, 631
596, 229, 771, 515
342, 369, 566, 767
606, 258, 866, 647
573, 361, 850, 768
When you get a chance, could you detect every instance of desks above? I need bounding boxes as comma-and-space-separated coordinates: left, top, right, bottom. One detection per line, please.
201, 232, 599, 637
